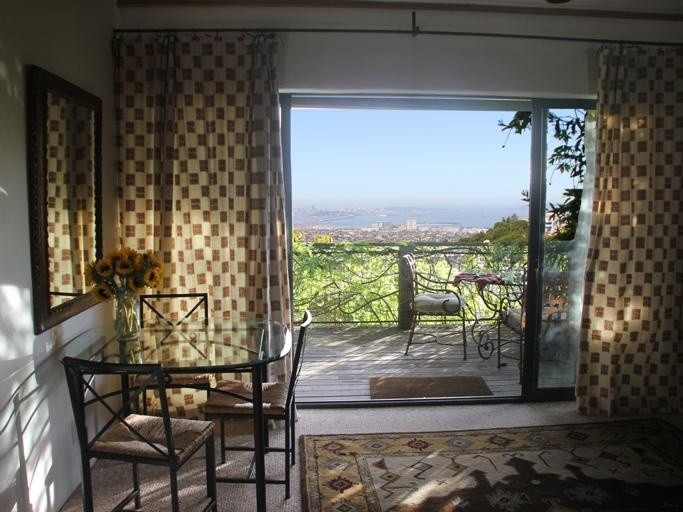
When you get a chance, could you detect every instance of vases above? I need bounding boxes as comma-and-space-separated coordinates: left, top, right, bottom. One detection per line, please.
113, 295, 140, 340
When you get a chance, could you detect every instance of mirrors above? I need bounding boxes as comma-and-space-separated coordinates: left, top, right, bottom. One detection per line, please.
22, 59, 106, 336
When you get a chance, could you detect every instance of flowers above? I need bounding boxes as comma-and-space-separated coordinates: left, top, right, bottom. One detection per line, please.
83, 248, 166, 335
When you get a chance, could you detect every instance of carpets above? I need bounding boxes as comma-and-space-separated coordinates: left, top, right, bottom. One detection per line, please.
297, 416, 683, 512
368, 372, 494, 400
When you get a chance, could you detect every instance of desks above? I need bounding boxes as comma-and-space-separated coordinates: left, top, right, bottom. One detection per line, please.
63, 318, 293, 497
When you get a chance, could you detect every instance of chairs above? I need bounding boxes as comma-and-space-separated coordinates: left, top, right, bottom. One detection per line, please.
140, 292, 211, 422
203, 308, 314, 500
64, 356, 218, 512
401, 252, 571, 387
